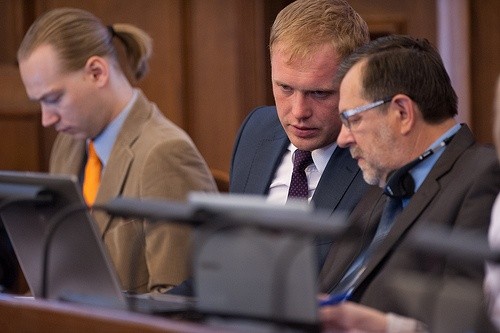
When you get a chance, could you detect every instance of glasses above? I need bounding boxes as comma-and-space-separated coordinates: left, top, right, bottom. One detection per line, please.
338, 97, 392, 129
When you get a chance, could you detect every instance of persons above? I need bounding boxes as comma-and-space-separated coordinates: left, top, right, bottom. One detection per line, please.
316, 33, 500, 333
229, 0, 373, 278
17, 7, 220, 295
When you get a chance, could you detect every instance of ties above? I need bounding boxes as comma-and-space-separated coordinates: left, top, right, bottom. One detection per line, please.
81, 141, 103, 208
329, 196, 404, 300
285, 149, 313, 209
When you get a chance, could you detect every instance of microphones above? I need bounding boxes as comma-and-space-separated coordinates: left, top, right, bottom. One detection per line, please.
40, 197, 210, 298
180, 205, 348, 322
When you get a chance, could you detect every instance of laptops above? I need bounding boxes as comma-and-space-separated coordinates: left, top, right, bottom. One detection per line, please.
186, 191, 318, 331
0, 171, 129, 312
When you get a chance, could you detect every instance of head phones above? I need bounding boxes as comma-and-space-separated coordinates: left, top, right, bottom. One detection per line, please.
383, 133, 456, 199
